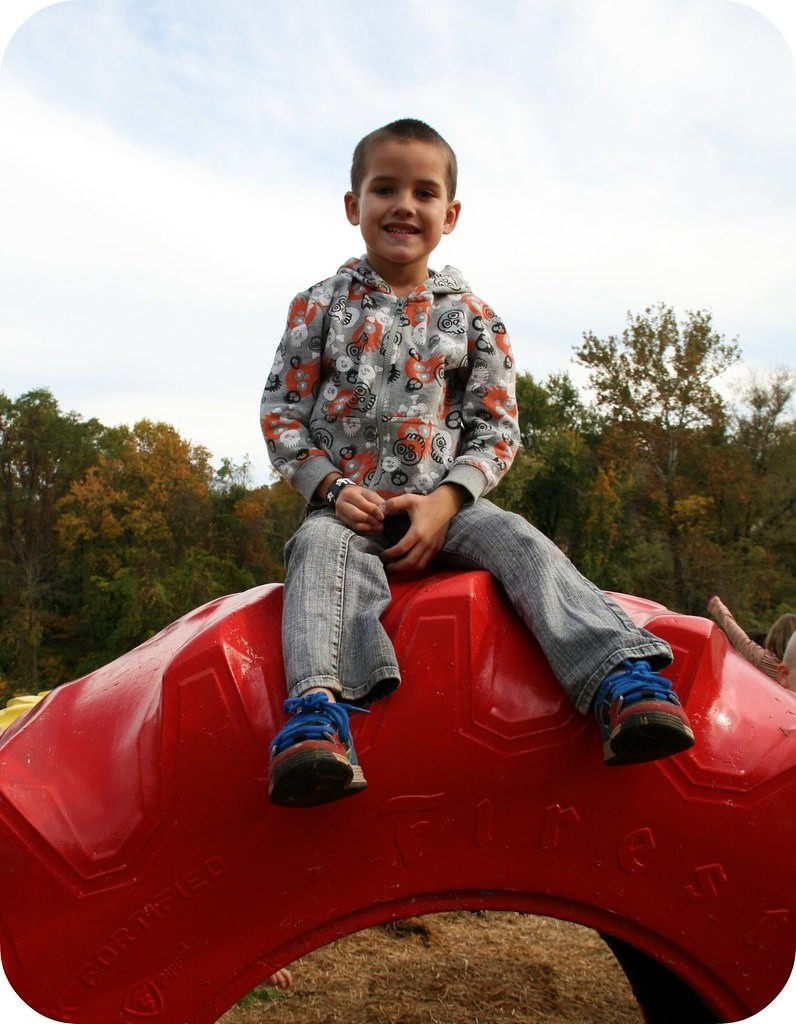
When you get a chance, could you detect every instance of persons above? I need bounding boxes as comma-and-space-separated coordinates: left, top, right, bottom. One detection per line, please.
260, 118, 694, 807
708, 596, 796, 694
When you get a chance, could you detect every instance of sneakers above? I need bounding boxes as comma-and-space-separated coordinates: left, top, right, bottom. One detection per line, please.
594, 658, 696, 766
268, 692, 370, 804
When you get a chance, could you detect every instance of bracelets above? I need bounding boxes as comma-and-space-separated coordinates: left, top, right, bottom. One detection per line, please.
326, 477, 356, 512
